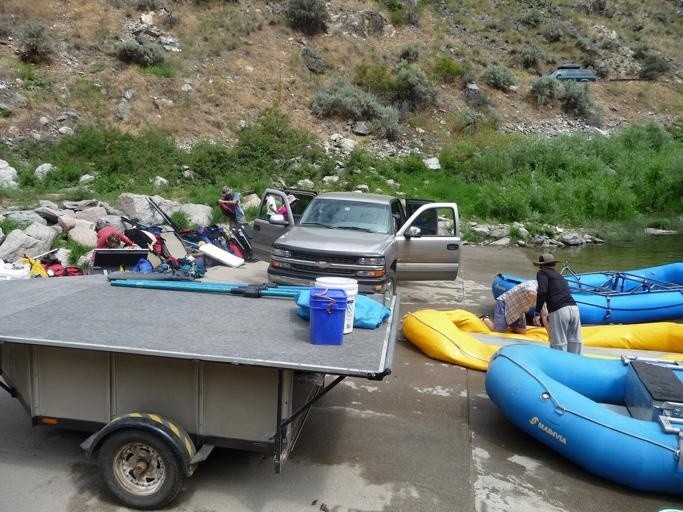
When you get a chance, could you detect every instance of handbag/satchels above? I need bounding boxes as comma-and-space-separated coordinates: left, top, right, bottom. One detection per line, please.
178, 224, 224, 242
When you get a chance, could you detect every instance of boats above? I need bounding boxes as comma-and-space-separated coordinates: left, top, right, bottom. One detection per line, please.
490, 262, 682, 325
483, 341, 682, 504
400, 307, 683, 374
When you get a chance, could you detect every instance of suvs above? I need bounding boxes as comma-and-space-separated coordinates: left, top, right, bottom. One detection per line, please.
252, 189, 462, 297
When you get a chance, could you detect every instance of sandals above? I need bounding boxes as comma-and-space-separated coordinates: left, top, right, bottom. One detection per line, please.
480, 314, 491, 322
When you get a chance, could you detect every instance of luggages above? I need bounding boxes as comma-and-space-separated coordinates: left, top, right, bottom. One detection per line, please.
199, 221, 257, 267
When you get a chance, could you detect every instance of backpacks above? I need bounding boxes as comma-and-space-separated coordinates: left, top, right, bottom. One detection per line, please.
131, 256, 208, 280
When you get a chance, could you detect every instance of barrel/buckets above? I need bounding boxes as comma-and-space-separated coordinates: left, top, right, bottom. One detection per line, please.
314, 276, 359, 334
309, 287, 348, 345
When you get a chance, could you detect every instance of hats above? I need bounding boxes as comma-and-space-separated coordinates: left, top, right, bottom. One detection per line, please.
284, 195, 301, 207
533, 254, 564, 266
221, 186, 233, 197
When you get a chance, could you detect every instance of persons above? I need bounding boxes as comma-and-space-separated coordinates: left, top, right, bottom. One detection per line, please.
266, 193, 281, 222
91, 226, 136, 263
277, 195, 297, 224
217, 186, 244, 225
535, 253, 582, 358
481, 281, 548, 335
